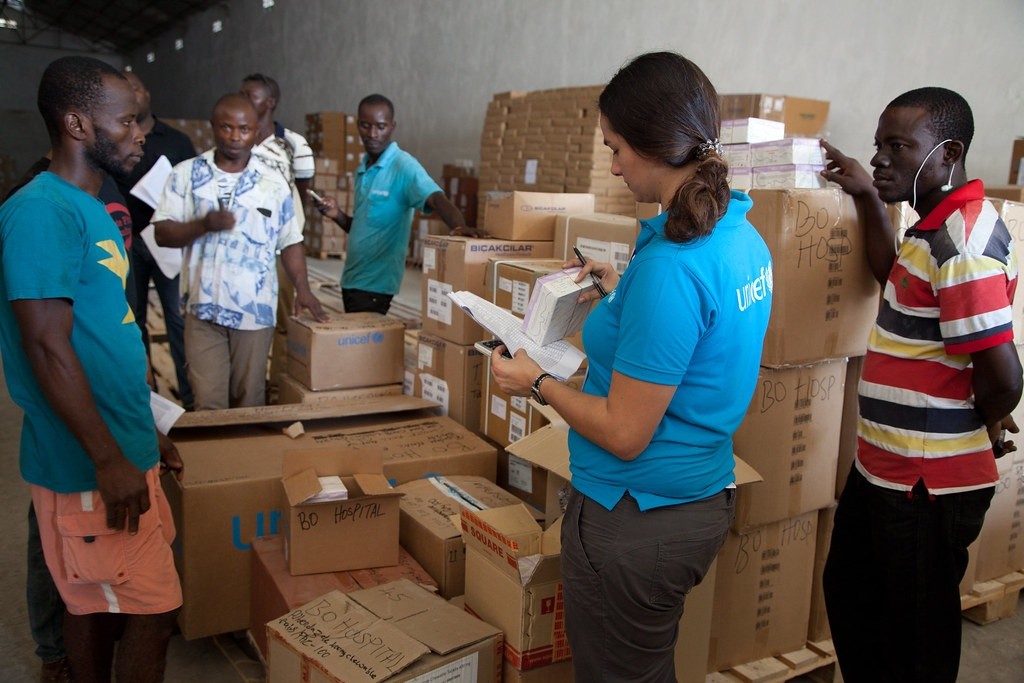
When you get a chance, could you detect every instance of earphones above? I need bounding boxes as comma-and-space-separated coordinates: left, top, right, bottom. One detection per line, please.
941, 184, 953, 191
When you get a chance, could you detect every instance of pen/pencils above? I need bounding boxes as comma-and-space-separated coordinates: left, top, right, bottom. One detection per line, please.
573, 245, 607, 298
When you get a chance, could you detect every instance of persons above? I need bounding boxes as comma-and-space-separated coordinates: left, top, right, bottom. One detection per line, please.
112, 72, 197, 411
0, 56, 183, 683
314, 94, 491, 314
0, 149, 138, 683
150, 96, 331, 412
241, 73, 315, 405
820, 86, 1024, 683
492, 52, 773, 682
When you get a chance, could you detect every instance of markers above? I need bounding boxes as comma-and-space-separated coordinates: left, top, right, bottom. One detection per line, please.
218, 198, 224, 209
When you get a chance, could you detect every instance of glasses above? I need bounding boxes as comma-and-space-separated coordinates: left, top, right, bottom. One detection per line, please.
243, 73, 275, 97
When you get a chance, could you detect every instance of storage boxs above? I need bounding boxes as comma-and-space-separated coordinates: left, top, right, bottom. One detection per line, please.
147, 80, 1024, 683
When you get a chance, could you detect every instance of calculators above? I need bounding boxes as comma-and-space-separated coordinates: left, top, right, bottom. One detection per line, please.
474, 339, 513, 360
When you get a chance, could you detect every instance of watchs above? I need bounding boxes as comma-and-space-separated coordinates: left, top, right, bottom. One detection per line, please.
531, 373, 557, 407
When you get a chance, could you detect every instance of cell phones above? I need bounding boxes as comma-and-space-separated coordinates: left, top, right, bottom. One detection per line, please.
474, 338, 512, 361
306, 189, 327, 206
